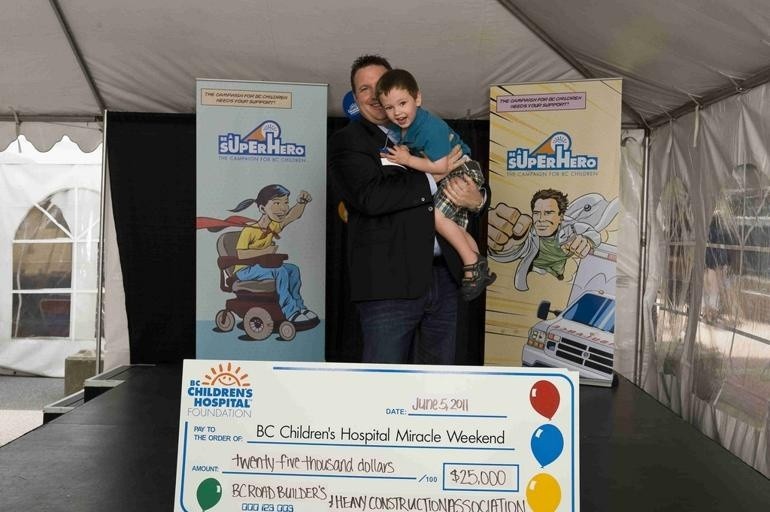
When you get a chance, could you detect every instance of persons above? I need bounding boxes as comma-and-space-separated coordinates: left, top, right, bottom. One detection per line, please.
337, 55, 462, 365
700, 211, 735, 322
375, 67, 499, 301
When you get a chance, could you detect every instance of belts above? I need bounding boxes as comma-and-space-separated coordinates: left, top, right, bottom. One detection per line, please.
433, 256, 445, 267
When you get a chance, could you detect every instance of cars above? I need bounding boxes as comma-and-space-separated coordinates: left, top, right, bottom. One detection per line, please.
521, 291, 614, 387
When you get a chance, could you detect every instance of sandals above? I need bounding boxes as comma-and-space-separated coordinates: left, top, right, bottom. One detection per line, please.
461, 252, 497, 301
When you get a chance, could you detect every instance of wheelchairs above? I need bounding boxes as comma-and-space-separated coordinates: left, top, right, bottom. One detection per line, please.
216, 231, 319, 340
